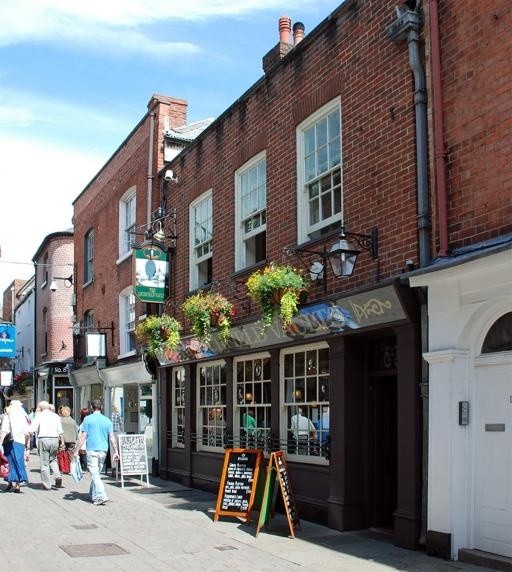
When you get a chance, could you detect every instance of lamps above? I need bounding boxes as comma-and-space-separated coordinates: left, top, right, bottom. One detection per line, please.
327, 219, 379, 279
164, 169, 179, 184
283, 244, 326, 293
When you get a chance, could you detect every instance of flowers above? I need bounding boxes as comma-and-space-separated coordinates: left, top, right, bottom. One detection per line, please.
180, 289, 237, 345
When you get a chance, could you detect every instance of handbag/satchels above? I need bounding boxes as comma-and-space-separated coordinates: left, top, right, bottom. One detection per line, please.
57, 450, 70, 474
68, 456, 83, 483
2, 432, 14, 456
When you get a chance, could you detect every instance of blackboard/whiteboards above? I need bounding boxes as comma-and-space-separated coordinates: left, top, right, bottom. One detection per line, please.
216, 448, 262, 517
274, 451, 300, 527
118, 434, 149, 475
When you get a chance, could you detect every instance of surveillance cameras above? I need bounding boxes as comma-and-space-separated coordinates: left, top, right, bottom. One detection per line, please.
165, 169, 177, 183
50, 280, 58, 293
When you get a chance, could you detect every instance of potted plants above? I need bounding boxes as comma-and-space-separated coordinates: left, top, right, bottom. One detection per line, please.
245, 262, 310, 336
133, 308, 179, 360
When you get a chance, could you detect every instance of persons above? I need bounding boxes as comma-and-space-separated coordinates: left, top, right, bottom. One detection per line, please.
72, 397, 120, 505
193, 389, 330, 456
1, 399, 91, 493
112, 404, 124, 432
1, 330, 11, 339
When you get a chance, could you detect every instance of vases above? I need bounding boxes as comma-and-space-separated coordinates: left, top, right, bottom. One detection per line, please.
210, 312, 223, 326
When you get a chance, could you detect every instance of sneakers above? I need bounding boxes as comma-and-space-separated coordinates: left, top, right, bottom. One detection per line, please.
55, 477, 62, 488
6, 485, 13, 492
41, 485, 48, 490
93, 498, 108, 505
15, 484, 20, 492
